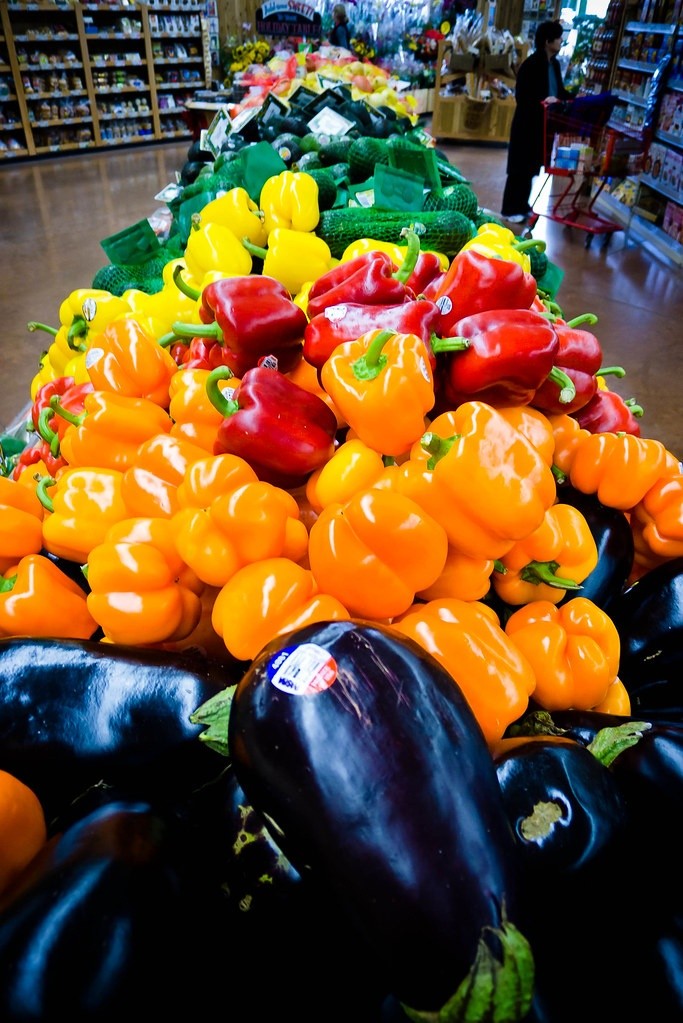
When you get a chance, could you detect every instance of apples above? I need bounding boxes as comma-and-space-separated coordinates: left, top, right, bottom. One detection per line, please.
230, 62, 416, 119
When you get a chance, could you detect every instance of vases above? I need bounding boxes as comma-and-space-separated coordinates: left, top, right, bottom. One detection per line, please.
401, 87, 445, 114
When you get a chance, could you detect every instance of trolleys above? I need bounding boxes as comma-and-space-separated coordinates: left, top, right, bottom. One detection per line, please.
521, 99, 648, 258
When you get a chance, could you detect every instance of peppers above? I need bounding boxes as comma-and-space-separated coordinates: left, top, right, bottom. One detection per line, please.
0, 230, 683, 889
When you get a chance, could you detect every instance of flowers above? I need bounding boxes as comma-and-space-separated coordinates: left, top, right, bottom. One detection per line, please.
346, 24, 448, 88
222, 40, 272, 89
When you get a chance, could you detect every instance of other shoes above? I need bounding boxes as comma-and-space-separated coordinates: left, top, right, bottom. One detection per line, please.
523, 211, 533, 217
500, 212, 524, 223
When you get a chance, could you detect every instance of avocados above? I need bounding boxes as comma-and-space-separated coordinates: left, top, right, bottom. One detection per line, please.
89, 136, 549, 296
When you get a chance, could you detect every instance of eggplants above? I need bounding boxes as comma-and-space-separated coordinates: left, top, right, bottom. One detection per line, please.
0, 489, 683, 1023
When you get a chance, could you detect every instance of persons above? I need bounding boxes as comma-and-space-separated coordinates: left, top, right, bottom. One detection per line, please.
316, 4, 350, 50
501, 20, 589, 226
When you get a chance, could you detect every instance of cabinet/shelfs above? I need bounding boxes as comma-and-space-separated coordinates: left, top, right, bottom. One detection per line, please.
589, 0, 683, 282
0, 0, 217, 162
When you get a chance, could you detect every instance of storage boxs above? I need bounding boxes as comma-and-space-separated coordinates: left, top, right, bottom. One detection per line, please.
576, 147, 594, 174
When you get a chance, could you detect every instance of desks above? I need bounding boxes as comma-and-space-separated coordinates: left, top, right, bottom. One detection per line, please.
184, 97, 239, 146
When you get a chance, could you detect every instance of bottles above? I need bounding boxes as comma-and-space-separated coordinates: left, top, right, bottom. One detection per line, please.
577, 0, 623, 99
